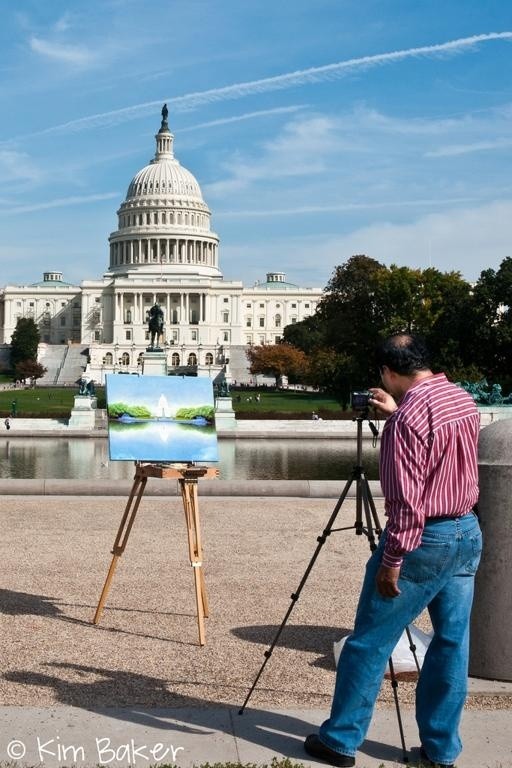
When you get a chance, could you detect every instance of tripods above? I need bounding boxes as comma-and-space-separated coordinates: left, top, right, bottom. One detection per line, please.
239, 421, 421, 763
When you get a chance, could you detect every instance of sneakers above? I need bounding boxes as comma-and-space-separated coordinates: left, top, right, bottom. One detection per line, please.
420, 744, 453, 768
304, 734, 355, 768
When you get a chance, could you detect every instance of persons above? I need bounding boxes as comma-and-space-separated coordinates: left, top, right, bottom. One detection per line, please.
148, 302, 164, 333
11, 401, 16, 417
311, 411, 318, 420
237, 392, 261, 403
304, 333, 484, 768
5, 419, 10, 430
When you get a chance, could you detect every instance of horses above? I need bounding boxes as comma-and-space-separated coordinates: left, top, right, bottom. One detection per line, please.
149, 309, 162, 349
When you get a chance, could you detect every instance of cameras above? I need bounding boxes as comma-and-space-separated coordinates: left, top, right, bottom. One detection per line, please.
350, 390, 372, 410
